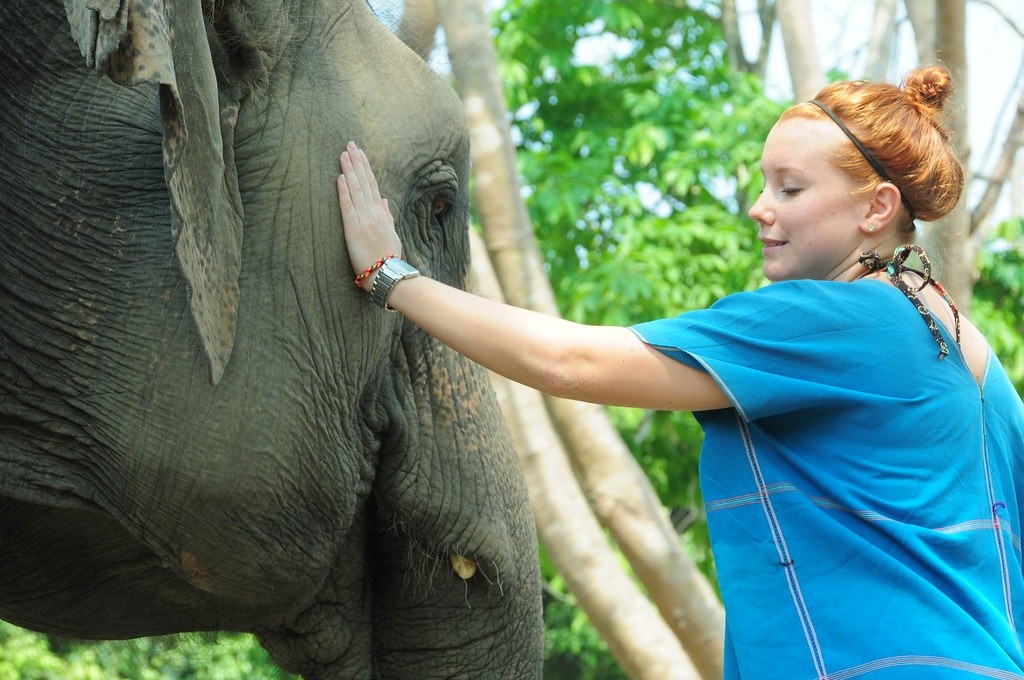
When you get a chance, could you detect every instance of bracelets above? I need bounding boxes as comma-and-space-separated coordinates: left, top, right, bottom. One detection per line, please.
355, 255, 402, 286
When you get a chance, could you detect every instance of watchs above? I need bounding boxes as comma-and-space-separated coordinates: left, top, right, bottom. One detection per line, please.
370, 259, 422, 313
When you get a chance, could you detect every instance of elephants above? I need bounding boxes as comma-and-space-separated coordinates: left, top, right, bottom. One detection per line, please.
0, 0, 543, 680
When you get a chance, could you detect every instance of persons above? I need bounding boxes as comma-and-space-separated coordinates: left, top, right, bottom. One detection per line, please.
335, 65, 1024, 679
